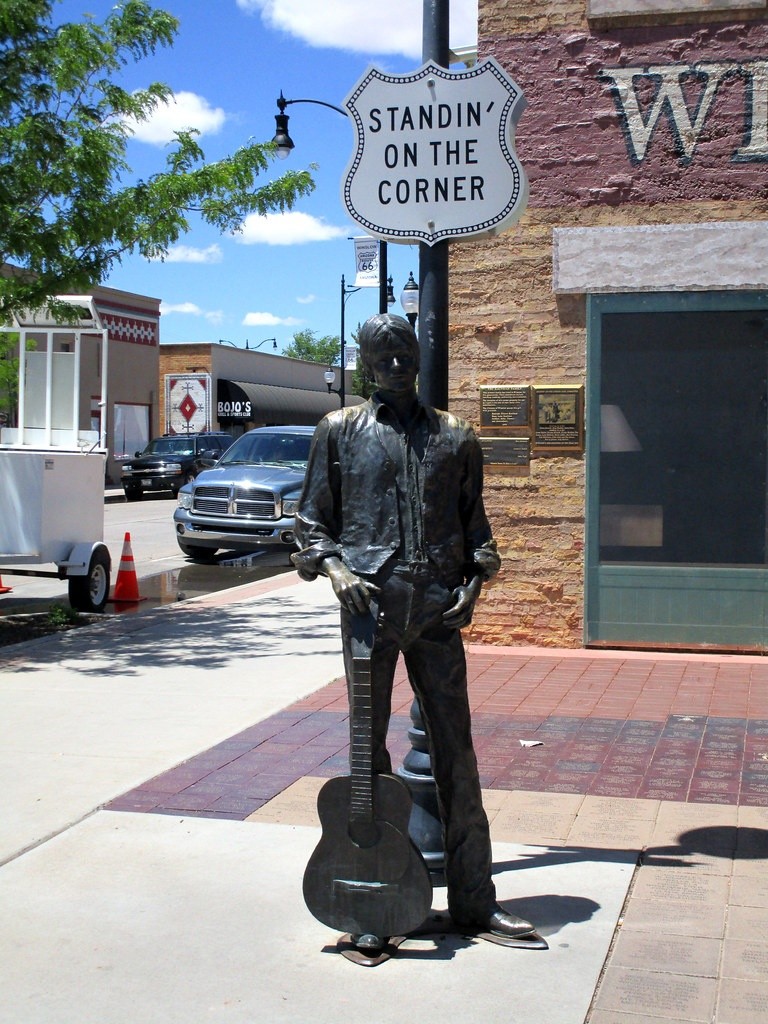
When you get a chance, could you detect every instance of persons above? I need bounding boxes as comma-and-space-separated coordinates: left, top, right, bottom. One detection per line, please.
288, 311, 537, 950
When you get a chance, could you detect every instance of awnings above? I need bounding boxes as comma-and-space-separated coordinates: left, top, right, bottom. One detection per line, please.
217, 378, 368, 425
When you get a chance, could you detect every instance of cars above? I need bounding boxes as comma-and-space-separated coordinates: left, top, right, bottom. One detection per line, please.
173, 425, 317, 560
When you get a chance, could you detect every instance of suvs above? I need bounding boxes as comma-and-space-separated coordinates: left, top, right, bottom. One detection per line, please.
120, 431, 236, 502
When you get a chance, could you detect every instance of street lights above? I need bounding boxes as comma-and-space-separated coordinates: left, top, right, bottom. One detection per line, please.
273, 90, 388, 315
323, 275, 395, 408
219, 337, 277, 352
400, 272, 419, 331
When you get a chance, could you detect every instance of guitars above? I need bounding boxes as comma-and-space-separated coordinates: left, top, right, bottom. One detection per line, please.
303, 597, 431, 932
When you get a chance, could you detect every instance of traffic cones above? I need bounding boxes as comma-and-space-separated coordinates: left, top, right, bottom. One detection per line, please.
107, 531, 148, 603
0, 574, 12, 592
